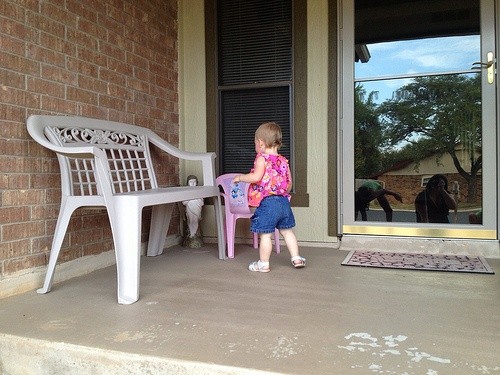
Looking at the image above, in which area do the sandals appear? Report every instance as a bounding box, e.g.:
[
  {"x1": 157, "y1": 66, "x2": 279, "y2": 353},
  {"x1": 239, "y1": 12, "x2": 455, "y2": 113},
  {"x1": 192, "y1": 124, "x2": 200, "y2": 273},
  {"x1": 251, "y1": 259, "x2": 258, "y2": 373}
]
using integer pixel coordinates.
[
  {"x1": 291, "y1": 256, "x2": 305, "y2": 267},
  {"x1": 248, "y1": 260, "x2": 269, "y2": 272}
]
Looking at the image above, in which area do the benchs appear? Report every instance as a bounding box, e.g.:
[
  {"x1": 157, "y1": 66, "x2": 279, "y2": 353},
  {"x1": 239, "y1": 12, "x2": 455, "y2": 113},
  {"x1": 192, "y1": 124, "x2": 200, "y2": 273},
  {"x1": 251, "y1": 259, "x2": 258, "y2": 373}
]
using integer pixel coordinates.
[{"x1": 26, "y1": 115, "x2": 229, "y2": 305}]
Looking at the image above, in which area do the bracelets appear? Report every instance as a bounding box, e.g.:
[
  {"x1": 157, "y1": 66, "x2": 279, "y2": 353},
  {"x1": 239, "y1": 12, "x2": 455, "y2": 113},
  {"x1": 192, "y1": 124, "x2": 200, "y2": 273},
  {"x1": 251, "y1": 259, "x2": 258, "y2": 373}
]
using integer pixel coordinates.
[{"x1": 238, "y1": 175, "x2": 242, "y2": 182}]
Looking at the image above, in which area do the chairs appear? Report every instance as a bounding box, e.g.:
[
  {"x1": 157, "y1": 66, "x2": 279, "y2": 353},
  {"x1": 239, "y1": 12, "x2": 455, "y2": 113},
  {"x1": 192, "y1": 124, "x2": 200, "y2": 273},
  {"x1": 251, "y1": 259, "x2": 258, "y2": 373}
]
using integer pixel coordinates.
[{"x1": 216, "y1": 173, "x2": 281, "y2": 259}]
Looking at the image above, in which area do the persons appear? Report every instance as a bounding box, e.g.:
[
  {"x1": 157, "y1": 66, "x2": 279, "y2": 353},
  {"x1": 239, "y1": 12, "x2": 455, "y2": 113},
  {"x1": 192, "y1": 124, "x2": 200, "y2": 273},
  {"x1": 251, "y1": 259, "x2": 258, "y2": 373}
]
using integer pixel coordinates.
[
  {"x1": 182, "y1": 175, "x2": 204, "y2": 237},
  {"x1": 233, "y1": 123, "x2": 306, "y2": 272},
  {"x1": 355, "y1": 174, "x2": 457, "y2": 224}
]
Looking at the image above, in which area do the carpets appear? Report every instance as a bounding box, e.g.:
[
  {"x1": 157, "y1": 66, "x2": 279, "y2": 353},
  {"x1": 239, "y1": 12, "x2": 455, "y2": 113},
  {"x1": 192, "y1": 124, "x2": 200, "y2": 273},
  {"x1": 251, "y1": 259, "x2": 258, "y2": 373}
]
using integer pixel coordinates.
[{"x1": 341, "y1": 250, "x2": 494, "y2": 275}]
